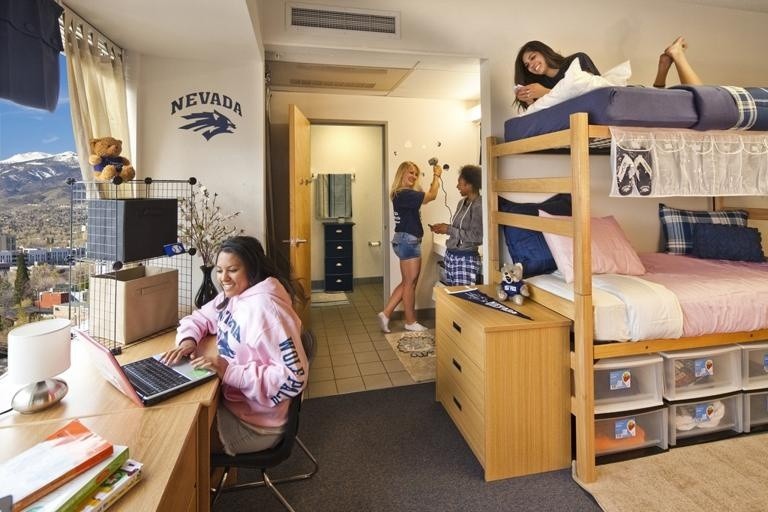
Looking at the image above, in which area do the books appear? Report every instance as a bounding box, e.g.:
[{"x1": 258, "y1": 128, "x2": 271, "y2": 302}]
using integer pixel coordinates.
[{"x1": 1, "y1": 413, "x2": 145, "y2": 512}]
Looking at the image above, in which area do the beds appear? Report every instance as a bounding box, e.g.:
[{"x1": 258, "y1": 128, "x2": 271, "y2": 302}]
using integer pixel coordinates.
[{"x1": 482, "y1": 84, "x2": 767, "y2": 482}]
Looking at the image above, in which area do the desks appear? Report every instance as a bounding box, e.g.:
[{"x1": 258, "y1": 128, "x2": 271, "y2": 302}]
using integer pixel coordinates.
[
  {"x1": 0, "y1": 400, "x2": 205, "y2": 512},
  {"x1": 0, "y1": 312, "x2": 229, "y2": 512}
]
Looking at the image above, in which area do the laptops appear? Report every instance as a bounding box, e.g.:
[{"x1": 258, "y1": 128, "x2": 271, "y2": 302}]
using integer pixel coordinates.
[{"x1": 72, "y1": 327, "x2": 218, "y2": 409}]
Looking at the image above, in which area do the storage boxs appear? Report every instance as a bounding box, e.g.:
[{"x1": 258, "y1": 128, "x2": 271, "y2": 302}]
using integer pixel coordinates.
[
  {"x1": 569, "y1": 345, "x2": 668, "y2": 413},
  {"x1": 78, "y1": 196, "x2": 182, "y2": 262},
  {"x1": 743, "y1": 389, "x2": 767, "y2": 435},
  {"x1": 743, "y1": 342, "x2": 767, "y2": 396},
  {"x1": 575, "y1": 401, "x2": 671, "y2": 458},
  {"x1": 670, "y1": 391, "x2": 746, "y2": 444},
  {"x1": 654, "y1": 345, "x2": 744, "y2": 403},
  {"x1": 85, "y1": 267, "x2": 180, "y2": 345}
]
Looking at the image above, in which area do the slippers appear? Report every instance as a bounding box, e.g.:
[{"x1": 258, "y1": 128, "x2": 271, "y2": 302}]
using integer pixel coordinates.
[{"x1": 615, "y1": 145, "x2": 653, "y2": 195}]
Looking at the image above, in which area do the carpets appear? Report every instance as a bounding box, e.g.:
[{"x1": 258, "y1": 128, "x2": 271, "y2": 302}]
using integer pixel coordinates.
[
  {"x1": 209, "y1": 376, "x2": 606, "y2": 512},
  {"x1": 571, "y1": 429, "x2": 767, "y2": 512},
  {"x1": 309, "y1": 287, "x2": 351, "y2": 308},
  {"x1": 383, "y1": 328, "x2": 440, "y2": 383}
]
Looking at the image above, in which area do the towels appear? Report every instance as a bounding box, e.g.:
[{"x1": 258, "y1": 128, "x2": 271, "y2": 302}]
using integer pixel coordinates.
[{"x1": 314, "y1": 172, "x2": 353, "y2": 220}]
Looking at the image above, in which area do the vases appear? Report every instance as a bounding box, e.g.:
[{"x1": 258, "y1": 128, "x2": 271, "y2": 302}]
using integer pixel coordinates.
[{"x1": 195, "y1": 262, "x2": 218, "y2": 311}]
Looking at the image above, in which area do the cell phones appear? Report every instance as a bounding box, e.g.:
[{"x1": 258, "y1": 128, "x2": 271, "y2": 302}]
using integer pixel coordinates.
[
  {"x1": 516, "y1": 84, "x2": 525, "y2": 91},
  {"x1": 428, "y1": 224, "x2": 433, "y2": 229}
]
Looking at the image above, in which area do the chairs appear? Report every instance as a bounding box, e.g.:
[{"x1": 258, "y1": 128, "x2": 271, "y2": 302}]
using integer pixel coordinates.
[{"x1": 207, "y1": 329, "x2": 320, "y2": 512}]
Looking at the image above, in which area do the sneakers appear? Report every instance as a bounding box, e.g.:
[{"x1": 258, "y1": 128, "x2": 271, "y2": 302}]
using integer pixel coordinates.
[
  {"x1": 404, "y1": 320, "x2": 428, "y2": 332},
  {"x1": 378, "y1": 312, "x2": 391, "y2": 333}
]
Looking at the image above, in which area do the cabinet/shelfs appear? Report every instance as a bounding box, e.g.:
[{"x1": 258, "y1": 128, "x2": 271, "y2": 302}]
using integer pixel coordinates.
[
  {"x1": 430, "y1": 281, "x2": 574, "y2": 482},
  {"x1": 66, "y1": 174, "x2": 196, "y2": 355},
  {"x1": 322, "y1": 220, "x2": 354, "y2": 295}
]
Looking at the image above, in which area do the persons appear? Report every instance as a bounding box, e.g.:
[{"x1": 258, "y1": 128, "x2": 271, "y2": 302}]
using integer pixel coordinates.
[
  {"x1": 157, "y1": 235, "x2": 310, "y2": 479},
  {"x1": 429, "y1": 165, "x2": 482, "y2": 287},
  {"x1": 377, "y1": 160, "x2": 442, "y2": 334},
  {"x1": 507, "y1": 35, "x2": 704, "y2": 110}
]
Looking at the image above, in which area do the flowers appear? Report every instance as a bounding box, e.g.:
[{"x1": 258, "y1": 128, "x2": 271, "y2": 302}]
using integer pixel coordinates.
[{"x1": 172, "y1": 180, "x2": 243, "y2": 269}]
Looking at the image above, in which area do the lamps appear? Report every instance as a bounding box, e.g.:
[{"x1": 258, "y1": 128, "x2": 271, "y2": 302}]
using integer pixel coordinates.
[{"x1": 5, "y1": 316, "x2": 73, "y2": 415}]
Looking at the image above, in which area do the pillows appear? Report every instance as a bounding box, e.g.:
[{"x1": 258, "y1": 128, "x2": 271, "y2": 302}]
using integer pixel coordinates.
[
  {"x1": 495, "y1": 191, "x2": 574, "y2": 280},
  {"x1": 693, "y1": 219, "x2": 766, "y2": 266},
  {"x1": 657, "y1": 202, "x2": 751, "y2": 255},
  {"x1": 518, "y1": 60, "x2": 634, "y2": 117},
  {"x1": 535, "y1": 210, "x2": 649, "y2": 281}
]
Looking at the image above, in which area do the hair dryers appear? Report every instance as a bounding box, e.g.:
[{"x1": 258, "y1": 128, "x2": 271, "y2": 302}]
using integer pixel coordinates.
[{"x1": 428, "y1": 158, "x2": 438, "y2": 167}]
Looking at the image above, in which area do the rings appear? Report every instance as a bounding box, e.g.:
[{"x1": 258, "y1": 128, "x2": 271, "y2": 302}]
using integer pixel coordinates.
[
  {"x1": 527, "y1": 94, "x2": 530, "y2": 98},
  {"x1": 439, "y1": 229, "x2": 440, "y2": 231}
]
